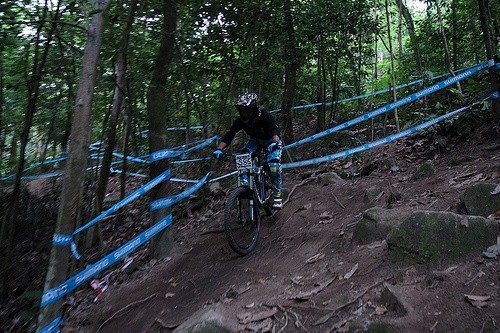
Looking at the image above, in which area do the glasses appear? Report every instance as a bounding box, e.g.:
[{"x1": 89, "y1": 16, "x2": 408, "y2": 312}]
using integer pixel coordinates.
[{"x1": 237, "y1": 106, "x2": 252, "y2": 113}]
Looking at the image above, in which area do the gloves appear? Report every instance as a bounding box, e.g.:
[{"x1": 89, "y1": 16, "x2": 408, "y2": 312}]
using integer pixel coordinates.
[
  {"x1": 262, "y1": 139, "x2": 281, "y2": 154},
  {"x1": 213, "y1": 150, "x2": 225, "y2": 160}
]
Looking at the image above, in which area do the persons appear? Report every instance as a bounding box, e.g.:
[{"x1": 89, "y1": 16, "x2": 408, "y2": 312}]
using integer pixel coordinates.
[{"x1": 210, "y1": 92, "x2": 285, "y2": 209}]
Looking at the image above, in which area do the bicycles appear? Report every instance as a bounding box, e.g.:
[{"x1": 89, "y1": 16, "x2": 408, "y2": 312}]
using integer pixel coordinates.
[{"x1": 216, "y1": 140, "x2": 278, "y2": 255}]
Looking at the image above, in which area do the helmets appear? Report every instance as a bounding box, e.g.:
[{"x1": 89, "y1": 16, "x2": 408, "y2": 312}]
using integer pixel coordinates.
[{"x1": 234, "y1": 92, "x2": 262, "y2": 125}]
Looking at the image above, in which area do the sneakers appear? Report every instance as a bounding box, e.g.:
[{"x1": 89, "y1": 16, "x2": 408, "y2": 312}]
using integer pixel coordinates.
[{"x1": 270, "y1": 197, "x2": 283, "y2": 209}]
